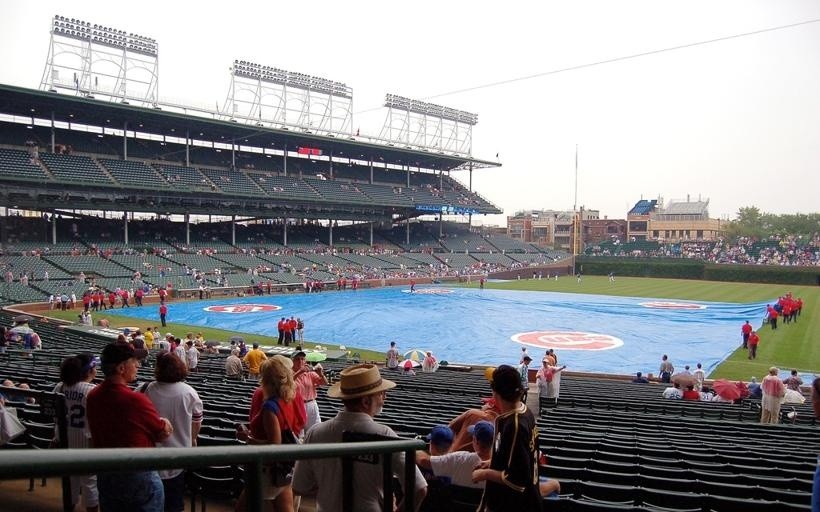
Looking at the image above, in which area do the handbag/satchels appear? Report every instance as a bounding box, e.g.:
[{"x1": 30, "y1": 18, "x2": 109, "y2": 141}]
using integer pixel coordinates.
[
  {"x1": 0, "y1": 402, "x2": 28, "y2": 445},
  {"x1": 263, "y1": 399, "x2": 300, "y2": 487}
]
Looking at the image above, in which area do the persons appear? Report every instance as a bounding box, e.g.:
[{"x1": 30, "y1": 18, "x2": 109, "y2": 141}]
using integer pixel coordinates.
[
  {"x1": 201, "y1": 175, "x2": 228, "y2": 189},
  {"x1": 592, "y1": 232, "x2": 820, "y2": 266},
  {"x1": 291, "y1": 172, "x2": 480, "y2": 205},
  {"x1": 0, "y1": 302, "x2": 567, "y2": 512},
  {"x1": 741, "y1": 320, "x2": 760, "y2": 360},
  {"x1": 518, "y1": 271, "x2": 616, "y2": 282},
  {"x1": 765, "y1": 292, "x2": 802, "y2": 330},
  {"x1": 223, "y1": 151, "x2": 283, "y2": 191},
  {"x1": 48, "y1": 143, "x2": 72, "y2": 154},
  {"x1": 633, "y1": 355, "x2": 802, "y2": 424},
  {"x1": 1, "y1": 211, "x2": 557, "y2": 312},
  {"x1": 28, "y1": 142, "x2": 39, "y2": 164},
  {"x1": 144, "y1": 159, "x2": 180, "y2": 181},
  {"x1": 811, "y1": 378, "x2": 820, "y2": 512}
]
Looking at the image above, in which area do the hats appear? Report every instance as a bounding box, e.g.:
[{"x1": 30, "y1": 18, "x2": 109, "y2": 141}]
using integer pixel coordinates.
[
  {"x1": 486, "y1": 365, "x2": 523, "y2": 393},
  {"x1": 426, "y1": 425, "x2": 454, "y2": 448},
  {"x1": 480, "y1": 398, "x2": 499, "y2": 411},
  {"x1": 524, "y1": 356, "x2": 532, "y2": 361},
  {"x1": 100, "y1": 343, "x2": 149, "y2": 367},
  {"x1": 291, "y1": 350, "x2": 306, "y2": 360},
  {"x1": 327, "y1": 363, "x2": 398, "y2": 400},
  {"x1": 542, "y1": 359, "x2": 549, "y2": 362},
  {"x1": 89, "y1": 355, "x2": 101, "y2": 370},
  {"x1": 467, "y1": 423, "x2": 495, "y2": 443}
]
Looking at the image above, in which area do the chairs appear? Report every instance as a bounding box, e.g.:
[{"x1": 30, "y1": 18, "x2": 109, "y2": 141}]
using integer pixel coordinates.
[
  {"x1": 0, "y1": 307, "x2": 820, "y2": 510},
  {"x1": 0, "y1": 142, "x2": 571, "y2": 305}
]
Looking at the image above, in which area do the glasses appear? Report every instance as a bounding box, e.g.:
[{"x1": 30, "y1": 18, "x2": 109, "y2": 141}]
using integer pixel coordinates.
[{"x1": 542, "y1": 362, "x2": 547, "y2": 364}]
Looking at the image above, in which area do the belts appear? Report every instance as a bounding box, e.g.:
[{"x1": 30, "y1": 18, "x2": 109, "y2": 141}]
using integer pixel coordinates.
[{"x1": 304, "y1": 399, "x2": 314, "y2": 403}]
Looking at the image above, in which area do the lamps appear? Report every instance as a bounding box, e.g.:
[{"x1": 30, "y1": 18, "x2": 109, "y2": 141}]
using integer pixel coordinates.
[
  {"x1": 51, "y1": 15, "x2": 157, "y2": 59},
  {"x1": 383, "y1": 93, "x2": 478, "y2": 127},
  {"x1": 232, "y1": 58, "x2": 352, "y2": 101}
]
